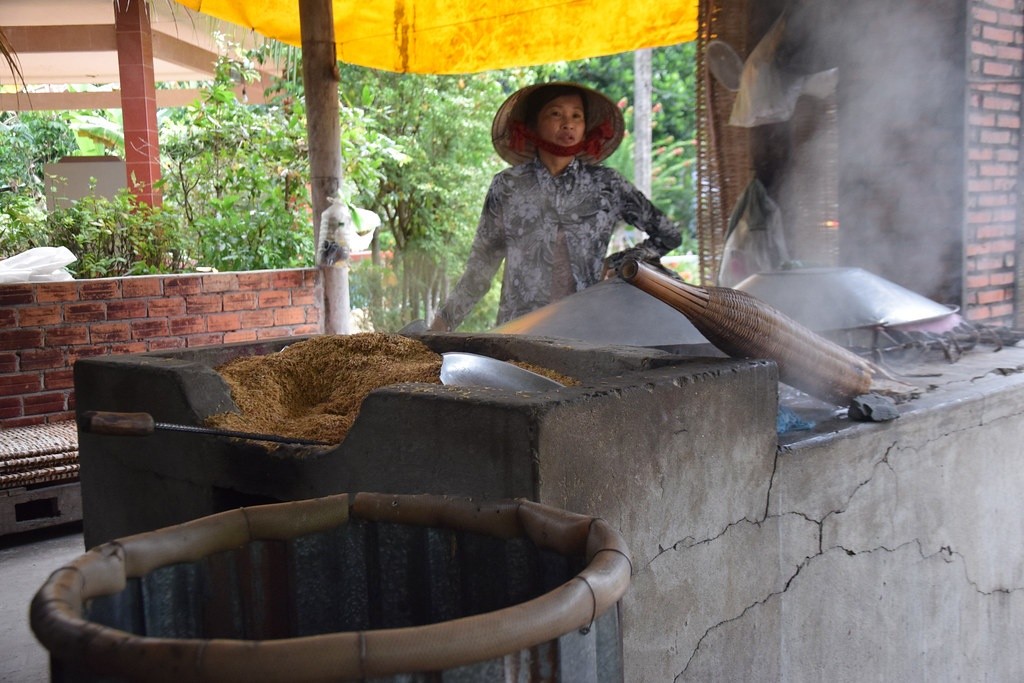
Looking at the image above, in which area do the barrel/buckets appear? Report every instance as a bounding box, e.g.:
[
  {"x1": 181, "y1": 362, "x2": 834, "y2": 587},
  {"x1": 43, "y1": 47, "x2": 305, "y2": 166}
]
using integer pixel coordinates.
[{"x1": 350, "y1": 209, "x2": 381, "y2": 255}]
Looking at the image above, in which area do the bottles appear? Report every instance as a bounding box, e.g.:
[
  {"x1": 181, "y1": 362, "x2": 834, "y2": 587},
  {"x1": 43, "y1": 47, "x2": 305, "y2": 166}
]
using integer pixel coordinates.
[
  {"x1": 334, "y1": 222, "x2": 351, "y2": 261},
  {"x1": 316, "y1": 197, "x2": 353, "y2": 267}
]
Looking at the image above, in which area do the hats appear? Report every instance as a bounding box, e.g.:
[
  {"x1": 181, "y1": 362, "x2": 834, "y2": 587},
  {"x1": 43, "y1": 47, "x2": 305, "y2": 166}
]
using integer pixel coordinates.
[{"x1": 492, "y1": 82, "x2": 625, "y2": 166}]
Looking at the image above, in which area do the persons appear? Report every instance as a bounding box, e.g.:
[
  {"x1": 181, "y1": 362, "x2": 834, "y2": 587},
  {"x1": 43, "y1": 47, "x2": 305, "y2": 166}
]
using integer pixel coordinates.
[{"x1": 429, "y1": 83, "x2": 685, "y2": 336}]
